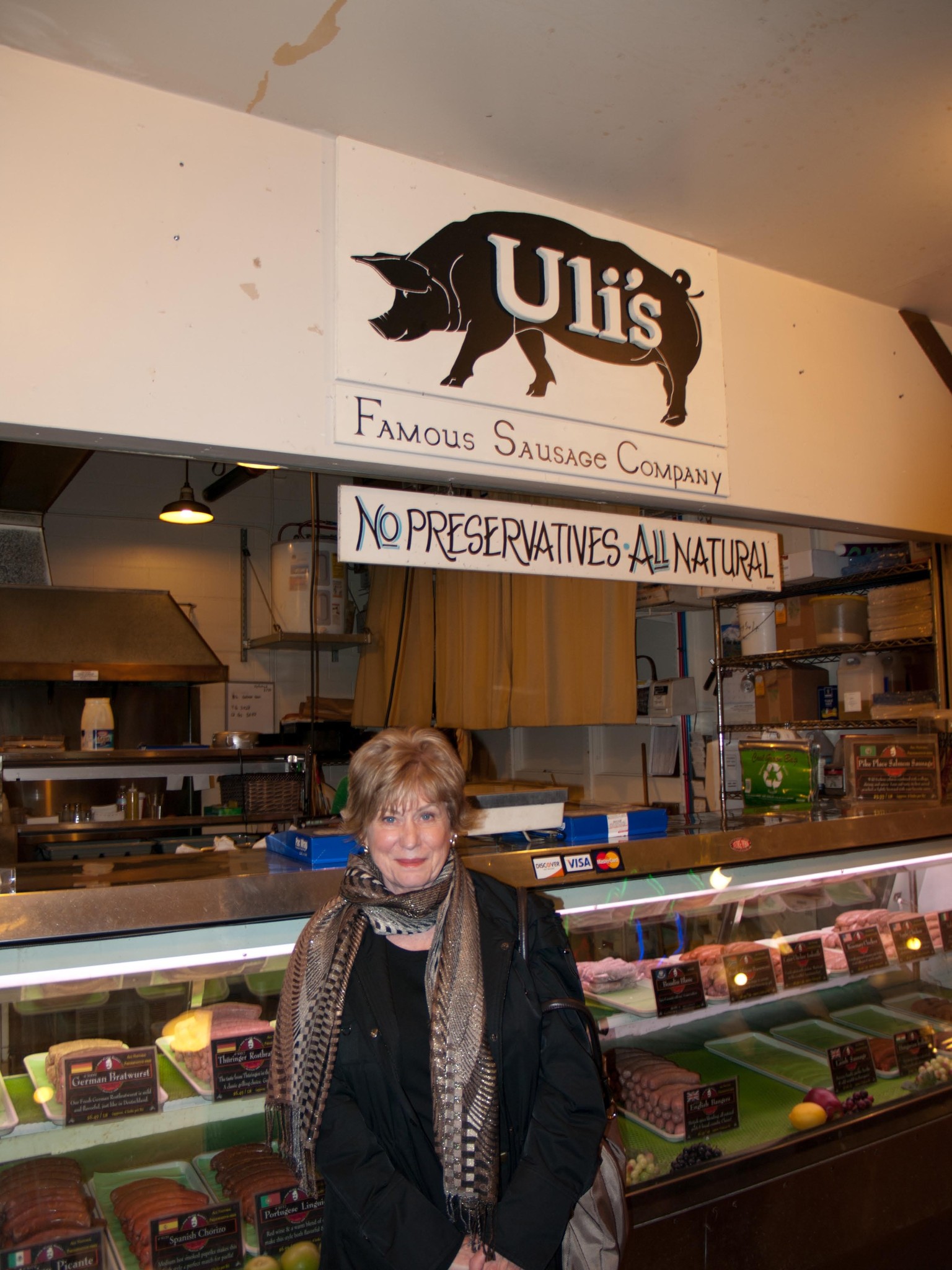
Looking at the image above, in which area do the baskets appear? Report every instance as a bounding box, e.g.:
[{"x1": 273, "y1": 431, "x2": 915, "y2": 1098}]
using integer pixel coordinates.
[{"x1": 216, "y1": 772, "x2": 304, "y2": 815}]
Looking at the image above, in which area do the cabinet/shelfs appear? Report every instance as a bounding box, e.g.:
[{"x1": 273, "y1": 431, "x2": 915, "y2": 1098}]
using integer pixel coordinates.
[{"x1": 0, "y1": 793, "x2": 950, "y2": 1268}]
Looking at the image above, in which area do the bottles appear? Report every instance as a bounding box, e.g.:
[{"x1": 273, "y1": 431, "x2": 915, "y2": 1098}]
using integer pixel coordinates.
[
  {"x1": 81, "y1": 698, "x2": 115, "y2": 751},
  {"x1": 116, "y1": 783, "x2": 139, "y2": 821},
  {"x1": 61, "y1": 802, "x2": 91, "y2": 823}
]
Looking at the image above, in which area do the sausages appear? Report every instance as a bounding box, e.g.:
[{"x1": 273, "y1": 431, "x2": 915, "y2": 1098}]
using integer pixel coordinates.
[{"x1": 1, "y1": 905, "x2": 949, "y2": 1270}]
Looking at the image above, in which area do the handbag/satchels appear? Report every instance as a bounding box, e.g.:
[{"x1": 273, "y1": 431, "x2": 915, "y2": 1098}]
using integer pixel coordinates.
[{"x1": 561, "y1": 1096, "x2": 630, "y2": 1270}]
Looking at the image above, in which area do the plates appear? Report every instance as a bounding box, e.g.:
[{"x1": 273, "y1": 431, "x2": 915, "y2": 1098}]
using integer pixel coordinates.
[{"x1": 4, "y1": 740, "x2": 65, "y2": 753}]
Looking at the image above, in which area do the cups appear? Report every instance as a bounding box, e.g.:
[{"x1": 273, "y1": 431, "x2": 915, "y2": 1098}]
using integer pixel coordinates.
[{"x1": 138, "y1": 792, "x2": 145, "y2": 820}]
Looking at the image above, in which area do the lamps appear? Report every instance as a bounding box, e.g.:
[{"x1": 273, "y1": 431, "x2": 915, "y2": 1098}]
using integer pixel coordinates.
[{"x1": 159, "y1": 457, "x2": 217, "y2": 530}]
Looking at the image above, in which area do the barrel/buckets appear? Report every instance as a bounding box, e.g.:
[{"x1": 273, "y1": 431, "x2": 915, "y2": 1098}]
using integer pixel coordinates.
[
  {"x1": 838, "y1": 650, "x2": 905, "y2": 721},
  {"x1": 740, "y1": 601, "x2": 776, "y2": 655},
  {"x1": 761, "y1": 729, "x2": 867, "y2": 794}
]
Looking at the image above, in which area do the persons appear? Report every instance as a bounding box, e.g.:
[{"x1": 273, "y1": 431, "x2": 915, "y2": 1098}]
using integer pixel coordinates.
[{"x1": 262, "y1": 723, "x2": 609, "y2": 1270}]
